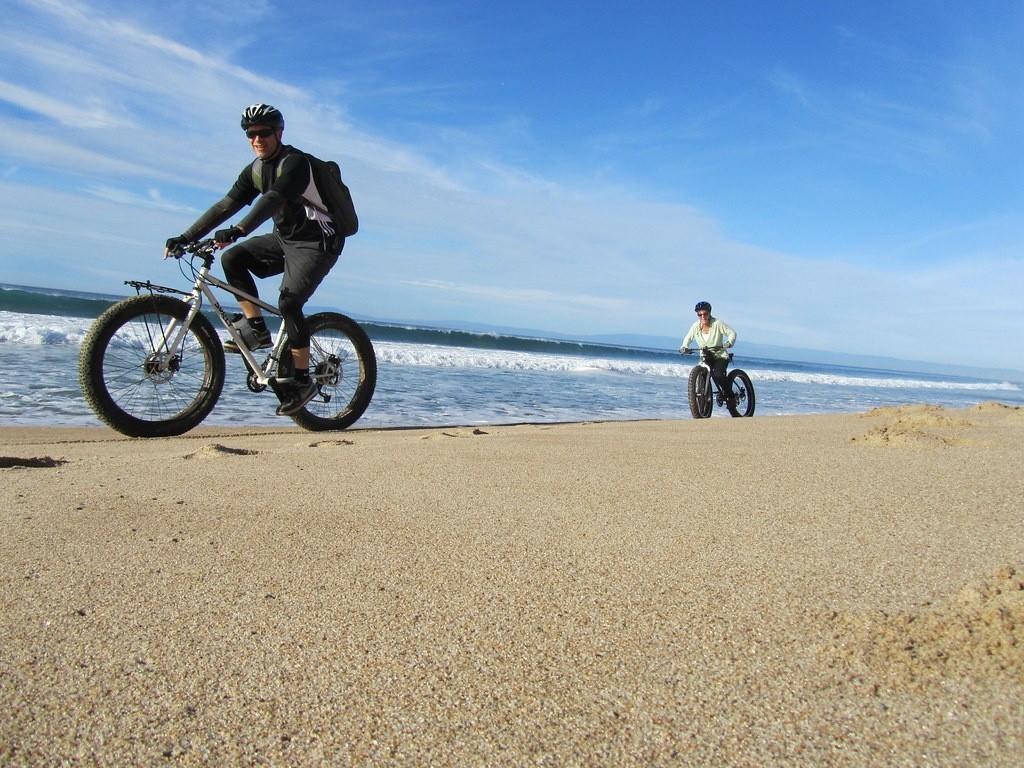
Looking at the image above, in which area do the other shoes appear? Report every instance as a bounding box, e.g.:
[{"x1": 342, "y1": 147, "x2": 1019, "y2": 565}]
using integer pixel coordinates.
[{"x1": 727, "y1": 392, "x2": 736, "y2": 410}]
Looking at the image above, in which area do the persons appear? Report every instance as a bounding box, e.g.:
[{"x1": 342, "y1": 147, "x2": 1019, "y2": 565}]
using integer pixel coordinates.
[
  {"x1": 680, "y1": 301, "x2": 737, "y2": 409},
  {"x1": 163, "y1": 104, "x2": 345, "y2": 416}
]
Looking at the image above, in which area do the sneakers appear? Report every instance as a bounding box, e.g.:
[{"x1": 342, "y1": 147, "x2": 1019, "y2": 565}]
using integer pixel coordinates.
[
  {"x1": 276, "y1": 378, "x2": 318, "y2": 415},
  {"x1": 223, "y1": 328, "x2": 274, "y2": 350}
]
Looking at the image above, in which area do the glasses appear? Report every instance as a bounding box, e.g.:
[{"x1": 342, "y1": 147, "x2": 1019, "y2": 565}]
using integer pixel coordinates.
[
  {"x1": 246, "y1": 128, "x2": 278, "y2": 139},
  {"x1": 697, "y1": 311, "x2": 709, "y2": 317}
]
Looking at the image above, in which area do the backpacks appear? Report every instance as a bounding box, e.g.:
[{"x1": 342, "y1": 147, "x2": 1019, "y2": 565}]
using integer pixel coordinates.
[{"x1": 252, "y1": 145, "x2": 359, "y2": 237}]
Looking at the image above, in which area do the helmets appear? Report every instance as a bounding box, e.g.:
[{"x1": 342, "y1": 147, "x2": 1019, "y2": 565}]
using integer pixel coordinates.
[
  {"x1": 694, "y1": 301, "x2": 711, "y2": 312},
  {"x1": 241, "y1": 103, "x2": 285, "y2": 130}
]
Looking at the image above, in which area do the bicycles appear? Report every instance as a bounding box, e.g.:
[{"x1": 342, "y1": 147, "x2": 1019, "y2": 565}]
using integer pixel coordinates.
[
  {"x1": 679, "y1": 344, "x2": 755, "y2": 419},
  {"x1": 79, "y1": 235, "x2": 378, "y2": 438}
]
